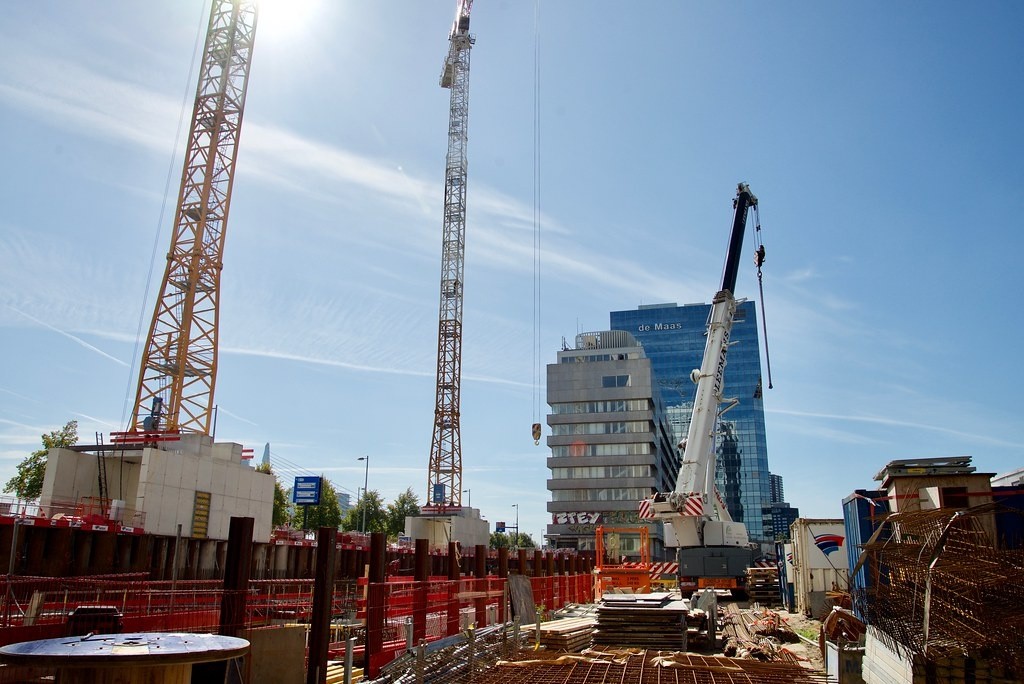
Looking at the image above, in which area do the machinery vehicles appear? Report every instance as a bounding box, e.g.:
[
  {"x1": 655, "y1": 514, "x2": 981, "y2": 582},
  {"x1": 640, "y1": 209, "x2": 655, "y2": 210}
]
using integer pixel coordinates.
[{"x1": 634, "y1": 181, "x2": 776, "y2": 601}]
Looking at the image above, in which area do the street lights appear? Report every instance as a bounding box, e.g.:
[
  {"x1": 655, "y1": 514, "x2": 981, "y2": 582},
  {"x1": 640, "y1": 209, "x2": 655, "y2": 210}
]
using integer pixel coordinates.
[
  {"x1": 512, "y1": 504, "x2": 520, "y2": 548},
  {"x1": 541, "y1": 527, "x2": 545, "y2": 549},
  {"x1": 358, "y1": 456, "x2": 369, "y2": 535},
  {"x1": 463, "y1": 489, "x2": 472, "y2": 508}
]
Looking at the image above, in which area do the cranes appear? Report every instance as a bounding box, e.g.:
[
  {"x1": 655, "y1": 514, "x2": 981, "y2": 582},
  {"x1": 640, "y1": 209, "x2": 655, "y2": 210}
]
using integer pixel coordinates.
[
  {"x1": 422, "y1": 1, "x2": 480, "y2": 510},
  {"x1": 119, "y1": 0, "x2": 264, "y2": 449}
]
